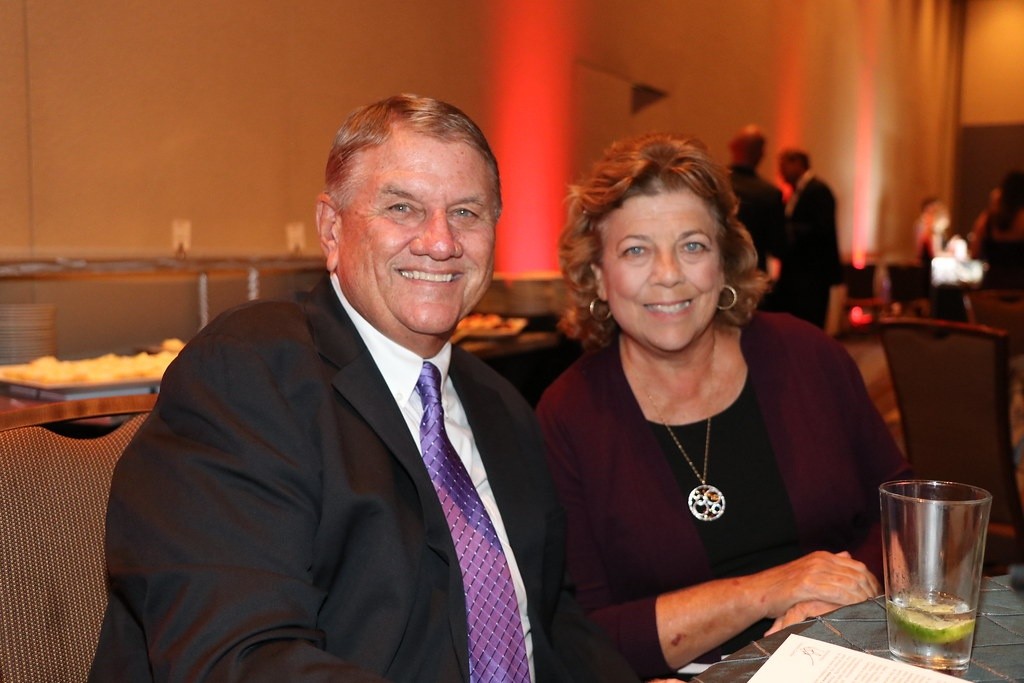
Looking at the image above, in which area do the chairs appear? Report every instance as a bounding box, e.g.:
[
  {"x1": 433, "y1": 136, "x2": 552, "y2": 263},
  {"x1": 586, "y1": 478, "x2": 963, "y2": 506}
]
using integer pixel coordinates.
[
  {"x1": 833, "y1": 290, "x2": 1024, "y2": 577},
  {"x1": 0, "y1": 394, "x2": 160, "y2": 683}
]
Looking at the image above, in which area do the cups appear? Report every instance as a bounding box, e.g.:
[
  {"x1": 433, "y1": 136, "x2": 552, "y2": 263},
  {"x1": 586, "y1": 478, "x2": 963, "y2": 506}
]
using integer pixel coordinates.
[{"x1": 880, "y1": 479, "x2": 993, "y2": 676}]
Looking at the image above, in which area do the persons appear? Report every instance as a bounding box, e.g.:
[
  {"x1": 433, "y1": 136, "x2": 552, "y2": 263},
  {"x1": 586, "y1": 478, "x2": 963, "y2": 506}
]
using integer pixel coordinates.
[
  {"x1": 534, "y1": 132, "x2": 906, "y2": 682},
  {"x1": 777, "y1": 146, "x2": 848, "y2": 338},
  {"x1": 721, "y1": 126, "x2": 785, "y2": 315},
  {"x1": 84, "y1": 92, "x2": 566, "y2": 683},
  {"x1": 916, "y1": 168, "x2": 1024, "y2": 289}
]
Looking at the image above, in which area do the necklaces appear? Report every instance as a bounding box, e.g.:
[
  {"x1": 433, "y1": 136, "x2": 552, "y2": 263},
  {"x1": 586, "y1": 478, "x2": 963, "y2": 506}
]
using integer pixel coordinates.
[{"x1": 633, "y1": 342, "x2": 726, "y2": 522}]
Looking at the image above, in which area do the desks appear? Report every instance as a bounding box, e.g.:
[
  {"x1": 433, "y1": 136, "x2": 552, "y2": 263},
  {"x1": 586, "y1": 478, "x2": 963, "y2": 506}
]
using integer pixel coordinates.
[{"x1": 688, "y1": 573, "x2": 1024, "y2": 683}]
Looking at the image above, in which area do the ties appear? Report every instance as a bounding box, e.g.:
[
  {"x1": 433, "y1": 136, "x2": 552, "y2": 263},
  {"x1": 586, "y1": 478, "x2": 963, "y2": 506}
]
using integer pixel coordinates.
[{"x1": 417, "y1": 361, "x2": 530, "y2": 683}]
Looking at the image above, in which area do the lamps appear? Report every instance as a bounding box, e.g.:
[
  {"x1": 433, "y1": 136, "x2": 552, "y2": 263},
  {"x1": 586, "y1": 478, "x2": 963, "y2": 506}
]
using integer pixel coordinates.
[{"x1": 568, "y1": 57, "x2": 669, "y2": 116}]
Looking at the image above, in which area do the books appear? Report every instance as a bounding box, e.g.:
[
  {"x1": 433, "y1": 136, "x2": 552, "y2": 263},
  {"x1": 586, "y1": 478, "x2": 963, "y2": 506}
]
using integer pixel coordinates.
[{"x1": 746, "y1": 633, "x2": 972, "y2": 683}]
